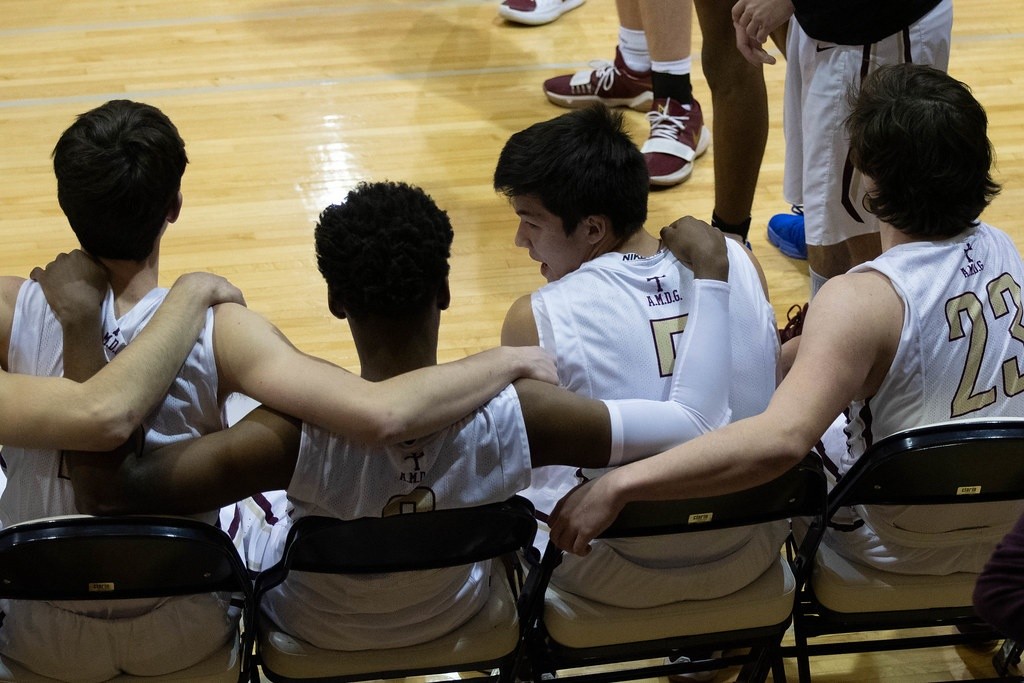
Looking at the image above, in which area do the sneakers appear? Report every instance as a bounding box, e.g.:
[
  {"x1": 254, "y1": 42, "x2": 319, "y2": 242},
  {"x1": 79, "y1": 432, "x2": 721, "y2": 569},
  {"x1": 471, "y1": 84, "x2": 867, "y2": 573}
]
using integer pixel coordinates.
[
  {"x1": 543, "y1": 46, "x2": 654, "y2": 111},
  {"x1": 499, "y1": 0, "x2": 586, "y2": 25},
  {"x1": 667, "y1": 650, "x2": 724, "y2": 682},
  {"x1": 637, "y1": 94, "x2": 710, "y2": 187},
  {"x1": 766, "y1": 205, "x2": 808, "y2": 259},
  {"x1": 775, "y1": 303, "x2": 809, "y2": 342}
]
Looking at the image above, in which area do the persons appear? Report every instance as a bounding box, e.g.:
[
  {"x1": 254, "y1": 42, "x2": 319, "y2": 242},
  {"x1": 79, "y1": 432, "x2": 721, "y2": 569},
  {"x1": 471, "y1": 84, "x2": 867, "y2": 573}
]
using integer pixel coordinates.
[
  {"x1": 30, "y1": 180, "x2": 732, "y2": 652},
  {"x1": 494, "y1": 103, "x2": 793, "y2": 683},
  {"x1": 972, "y1": 512, "x2": 1024, "y2": 644},
  {"x1": 0, "y1": 272, "x2": 249, "y2": 452},
  {"x1": 547, "y1": 62, "x2": 1024, "y2": 575},
  {"x1": 0, "y1": 99, "x2": 560, "y2": 683},
  {"x1": 497, "y1": 0, "x2": 954, "y2": 344}
]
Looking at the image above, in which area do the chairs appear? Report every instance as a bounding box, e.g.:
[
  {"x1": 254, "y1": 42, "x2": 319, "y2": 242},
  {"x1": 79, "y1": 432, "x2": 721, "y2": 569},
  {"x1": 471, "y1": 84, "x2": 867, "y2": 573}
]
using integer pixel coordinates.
[{"x1": 0, "y1": 415, "x2": 1024, "y2": 683}]
